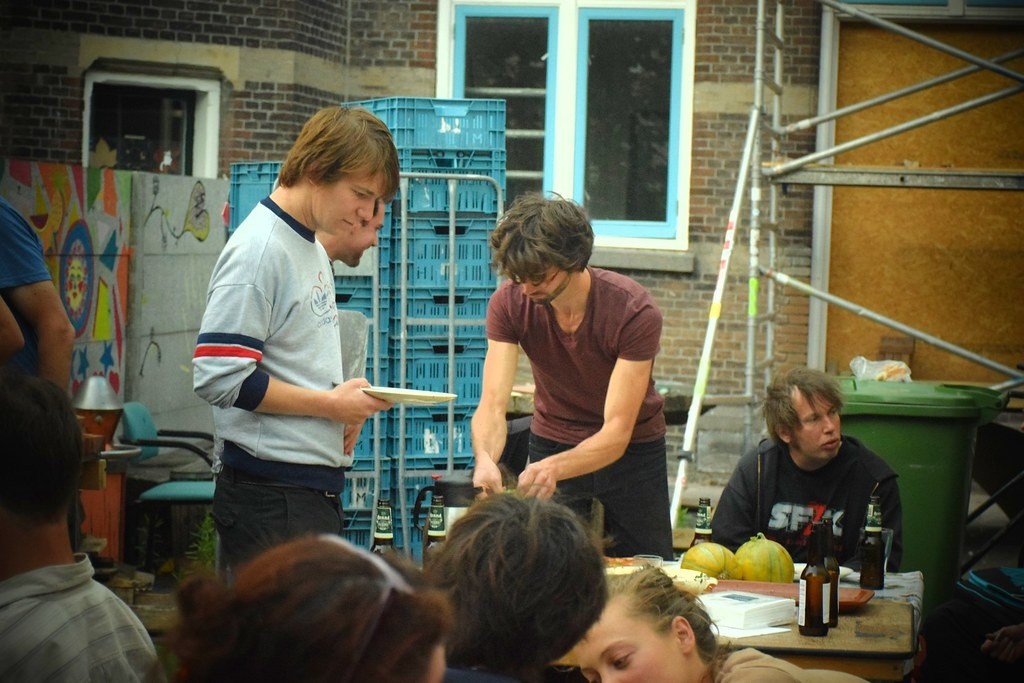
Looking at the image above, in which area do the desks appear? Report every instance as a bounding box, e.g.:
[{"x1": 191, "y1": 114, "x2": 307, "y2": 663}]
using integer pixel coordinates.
[{"x1": 550, "y1": 558, "x2": 923, "y2": 683}]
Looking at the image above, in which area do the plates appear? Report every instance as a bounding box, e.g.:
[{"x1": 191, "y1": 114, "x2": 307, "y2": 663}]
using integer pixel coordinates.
[
  {"x1": 712, "y1": 576, "x2": 876, "y2": 613},
  {"x1": 361, "y1": 386, "x2": 458, "y2": 406}
]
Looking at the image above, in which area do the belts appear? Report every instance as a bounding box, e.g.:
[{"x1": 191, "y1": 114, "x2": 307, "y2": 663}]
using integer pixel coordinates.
[{"x1": 219, "y1": 469, "x2": 342, "y2": 497}]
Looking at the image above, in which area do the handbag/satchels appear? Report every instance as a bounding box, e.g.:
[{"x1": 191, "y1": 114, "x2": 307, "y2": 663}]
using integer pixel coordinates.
[{"x1": 850, "y1": 356, "x2": 913, "y2": 383}]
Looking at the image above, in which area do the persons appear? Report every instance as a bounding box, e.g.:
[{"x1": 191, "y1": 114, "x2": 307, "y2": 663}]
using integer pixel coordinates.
[
  {"x1": 577, "y1": 568, "x2": 869, "y2": 683},
  {"x1": 0, "y1": 196, "x2": 75, "y2": 395},
  {"x1": 469, "y1": 193, "x2": 672, "y2": 560},
  {"x1": 312, "y1": 199, "x2": 385, "y2": 279},
  {"x1": 914, "y1": 598, "x2": 1024, "y2": 683},
  {"x1": 193, "y1": 105, "x2": 400, "y2": 580},
  {"x1": 0, "y1": 371, "x2": 164, "y2": 683},
  {"x1": 418, "y1": 492, "x2": 609, "y2": 683},
  {"x1": 159, "y1": 535, "x2": 449, "y2": 683},
  {"x1": 710, "y1": 365, "x2": 902, "y2": 569}
]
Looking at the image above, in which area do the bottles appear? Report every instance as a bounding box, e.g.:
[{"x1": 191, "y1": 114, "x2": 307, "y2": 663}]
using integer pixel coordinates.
[
  {"x1": 690, "y1": 497, "x2": 713, "y2": 547},
  {"x1": 821, "y1": 515, "x2": 840, "y2": 628},
  {"x1": 799, "y1": 521, "x2": 831, "y2": 637},
  {"x1": 422, "y1": 495, "x2": 447, "y2": 571},
  {"x1": 860, "y1": 495, "x2": 884, "y2": 590},
  {"x1": 369, "y1": 498, "x2": 401, "y2": 557}
]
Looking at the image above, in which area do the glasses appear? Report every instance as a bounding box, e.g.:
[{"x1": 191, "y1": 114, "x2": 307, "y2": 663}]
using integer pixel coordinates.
[{"x1": 507, "y1": 267, "x2": 560, "y2": 287}]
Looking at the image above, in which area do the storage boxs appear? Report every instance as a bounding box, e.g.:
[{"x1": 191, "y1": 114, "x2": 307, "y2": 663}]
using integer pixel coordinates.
[{"x1": 228, "y1": 97, "x2": 507, "y2": 570}]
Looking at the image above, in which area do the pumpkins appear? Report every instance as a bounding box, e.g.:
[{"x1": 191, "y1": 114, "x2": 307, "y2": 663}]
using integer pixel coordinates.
[{"x1": 682, "y1": 533, "x2": 796, "y2": 583}]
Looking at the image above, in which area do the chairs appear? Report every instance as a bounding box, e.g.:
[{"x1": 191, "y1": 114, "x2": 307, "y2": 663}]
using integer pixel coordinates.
[{"x1": 121, "y1": 401, "x2": 215, "y2": 569}]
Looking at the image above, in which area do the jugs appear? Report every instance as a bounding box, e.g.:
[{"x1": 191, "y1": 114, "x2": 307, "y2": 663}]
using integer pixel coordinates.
[{"x1": 414, "y1": 476, "x2": 484, "y2": 547}]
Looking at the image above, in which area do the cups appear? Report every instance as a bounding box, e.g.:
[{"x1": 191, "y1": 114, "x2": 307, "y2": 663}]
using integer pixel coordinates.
[
  {"x1": 858, "y1": 527, "x2": 894, "y2": 576},
  {"x1": 634, "y1": 554, "x2": 664, "y2": 569}
]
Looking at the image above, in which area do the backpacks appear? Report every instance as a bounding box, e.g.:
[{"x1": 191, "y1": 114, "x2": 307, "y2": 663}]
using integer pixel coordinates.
[
  {"x1": 957, "y1": 567, "x2": 1024, "y2": 625},
  {"x1": 922, "y1": 599, "x2": 1024, "y2": 683}
]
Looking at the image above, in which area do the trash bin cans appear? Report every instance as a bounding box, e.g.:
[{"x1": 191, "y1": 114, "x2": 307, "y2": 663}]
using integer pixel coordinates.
[{"x1": 833, "y1": 374, "x2": 1011, "y2": 599}]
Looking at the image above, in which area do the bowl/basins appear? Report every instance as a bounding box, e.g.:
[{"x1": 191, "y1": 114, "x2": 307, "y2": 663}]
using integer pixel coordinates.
[{"x1": 604, "y1": 567, "x2": 708, "y2": 596}]
